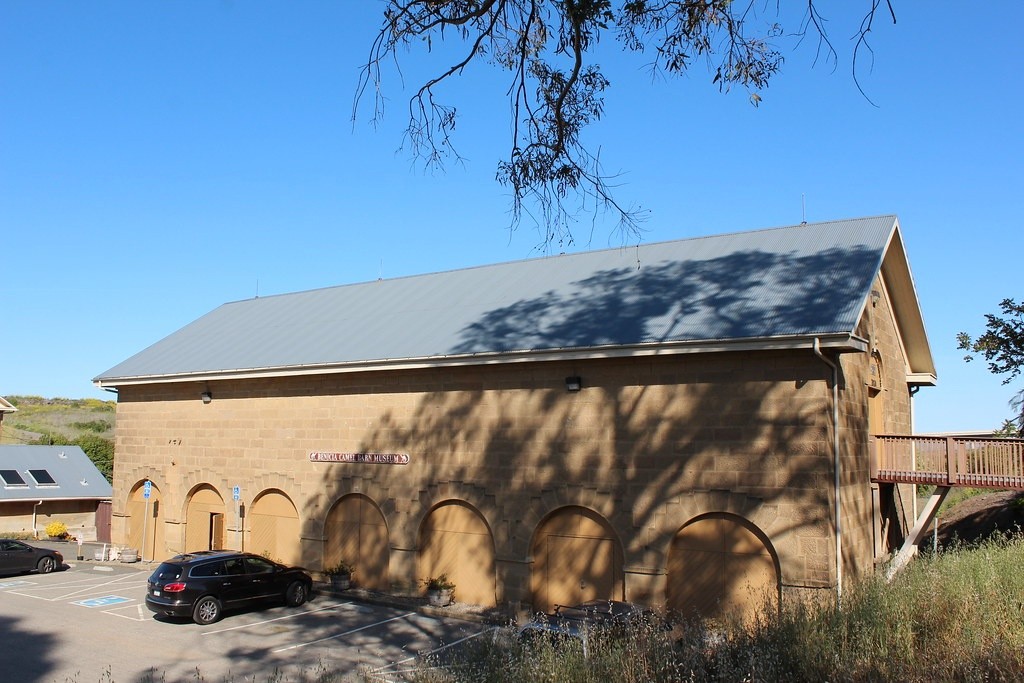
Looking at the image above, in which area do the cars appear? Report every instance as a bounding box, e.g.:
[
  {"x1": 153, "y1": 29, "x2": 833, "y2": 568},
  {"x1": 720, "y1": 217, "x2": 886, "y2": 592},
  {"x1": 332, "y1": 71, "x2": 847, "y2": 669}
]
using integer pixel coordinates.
[{"x1": 0, "y1": 539, "x2": 64, "y2": 578}]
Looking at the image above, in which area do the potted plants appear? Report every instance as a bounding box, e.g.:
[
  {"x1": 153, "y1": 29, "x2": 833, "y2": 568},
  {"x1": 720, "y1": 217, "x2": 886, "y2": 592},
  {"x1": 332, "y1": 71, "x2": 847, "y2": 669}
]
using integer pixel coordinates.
[
  {"x1": 326, "y1": 562, "x2": 355, "y2": 591},
  {"x1": 423, "y1": 574, "x2": 454, "y2": 607}
]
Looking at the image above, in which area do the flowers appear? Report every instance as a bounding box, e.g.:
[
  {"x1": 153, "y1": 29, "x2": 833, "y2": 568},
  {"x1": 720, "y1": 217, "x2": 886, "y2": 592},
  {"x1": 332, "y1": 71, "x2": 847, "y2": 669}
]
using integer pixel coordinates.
[{"x1": 45, "y1": 522, "x2": 67, "y2": 537}]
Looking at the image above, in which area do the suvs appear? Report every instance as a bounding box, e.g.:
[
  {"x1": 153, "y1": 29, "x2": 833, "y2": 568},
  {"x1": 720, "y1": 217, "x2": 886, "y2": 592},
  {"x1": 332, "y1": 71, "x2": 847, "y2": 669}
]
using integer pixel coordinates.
[{"x1": 145, "y1": 549, "x2": 313, "y2": 619}]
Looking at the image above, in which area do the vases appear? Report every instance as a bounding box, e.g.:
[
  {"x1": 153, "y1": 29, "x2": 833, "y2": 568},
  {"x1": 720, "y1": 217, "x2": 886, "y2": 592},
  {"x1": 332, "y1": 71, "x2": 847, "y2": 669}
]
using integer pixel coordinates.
[{"x1": 49, "y1": 534, "x2": 62, "y2": 541}]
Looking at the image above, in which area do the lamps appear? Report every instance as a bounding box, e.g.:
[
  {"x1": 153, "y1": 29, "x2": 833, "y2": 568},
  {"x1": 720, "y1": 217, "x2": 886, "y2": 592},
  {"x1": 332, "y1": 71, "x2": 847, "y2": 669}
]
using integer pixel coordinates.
[
  {"x1": 565, "y1": 376, "x2": 582, "y2": 391},
  {"x1": 202, "y1": 392, "x2": 212, "y2": 403},
  {"x1": 872, "y1": 290, "x2": 881, "y2": 302}
]
